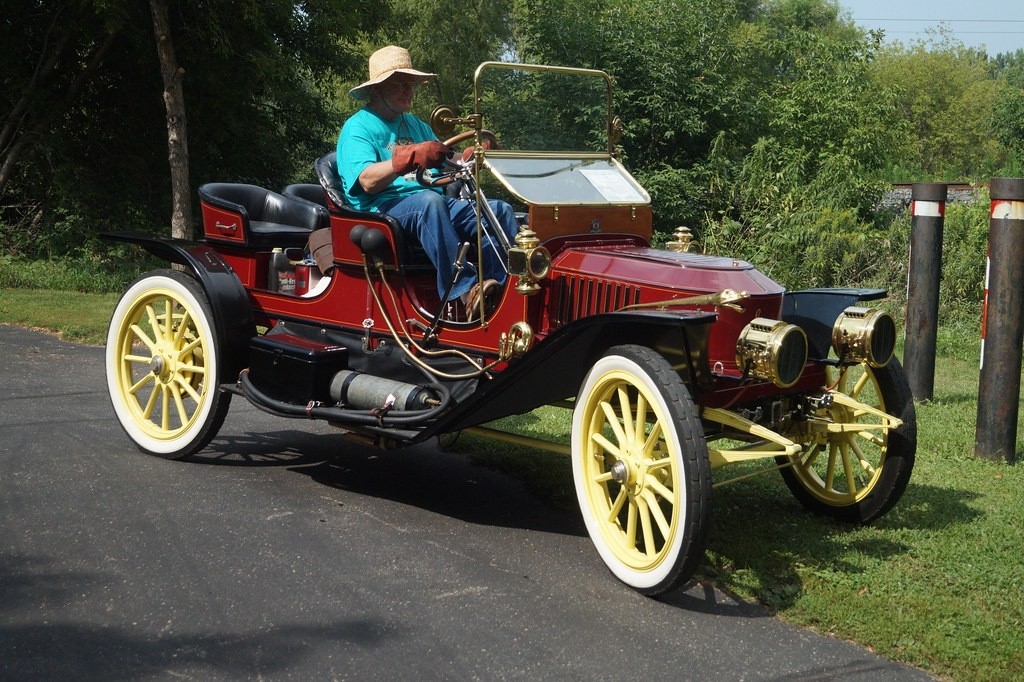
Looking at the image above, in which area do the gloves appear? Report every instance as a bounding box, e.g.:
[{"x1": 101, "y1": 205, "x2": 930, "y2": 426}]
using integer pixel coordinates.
[
  {"x1": 392, "y1": 140, "x2": 454, "y2": 177},
  {"x1": 463, "y1": 140, "x2": 499, "y2": 162}
]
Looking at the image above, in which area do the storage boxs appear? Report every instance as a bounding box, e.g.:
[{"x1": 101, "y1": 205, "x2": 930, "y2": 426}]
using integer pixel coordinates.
[{"x1": 249, "y1": 333, "x2": 349, "y2": 409}]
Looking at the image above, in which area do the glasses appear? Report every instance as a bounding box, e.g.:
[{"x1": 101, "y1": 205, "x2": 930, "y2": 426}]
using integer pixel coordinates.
[{"x1": 386, "y1": 79, "x2": 419, "y2": 93}]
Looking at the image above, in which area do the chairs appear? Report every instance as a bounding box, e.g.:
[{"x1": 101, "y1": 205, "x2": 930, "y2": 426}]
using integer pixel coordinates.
[
  {"x1": 282, "y1": 181, "x2": 335, "y2": 226},
  {"x1": 198, "y1": 182, "x2": 321, "y2": 246}
]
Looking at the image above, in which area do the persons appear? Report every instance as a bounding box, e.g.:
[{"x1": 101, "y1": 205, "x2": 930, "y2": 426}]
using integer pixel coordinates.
[{"x1": 335, "y1": 44, "x2": 520, "y2": 322}]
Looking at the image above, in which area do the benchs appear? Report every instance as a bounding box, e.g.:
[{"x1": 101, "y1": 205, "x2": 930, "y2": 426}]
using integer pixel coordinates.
[{"x1": 316, "y1": 150, "x2": 529, "y2": 267}]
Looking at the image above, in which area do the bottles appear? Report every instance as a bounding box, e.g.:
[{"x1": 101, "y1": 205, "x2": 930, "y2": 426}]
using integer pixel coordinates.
[{"x1": 268, "y1": 248, "x2": 289, "y2": 295}]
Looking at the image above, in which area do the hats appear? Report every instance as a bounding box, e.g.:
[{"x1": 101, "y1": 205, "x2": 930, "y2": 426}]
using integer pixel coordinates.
[{"x1": 349, "y1": 44, "x2": 438, "y2": 100}]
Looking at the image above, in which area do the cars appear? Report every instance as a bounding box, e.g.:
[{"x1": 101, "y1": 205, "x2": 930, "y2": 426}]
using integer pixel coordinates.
[{"x1": 107, "y1": 62, "x2": 919, "y2": 597}]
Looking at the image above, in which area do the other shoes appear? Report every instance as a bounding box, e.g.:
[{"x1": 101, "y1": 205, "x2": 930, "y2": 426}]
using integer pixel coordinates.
[{"x1": 465, "y1": 279, "x2": 499, "y2": 322}]
